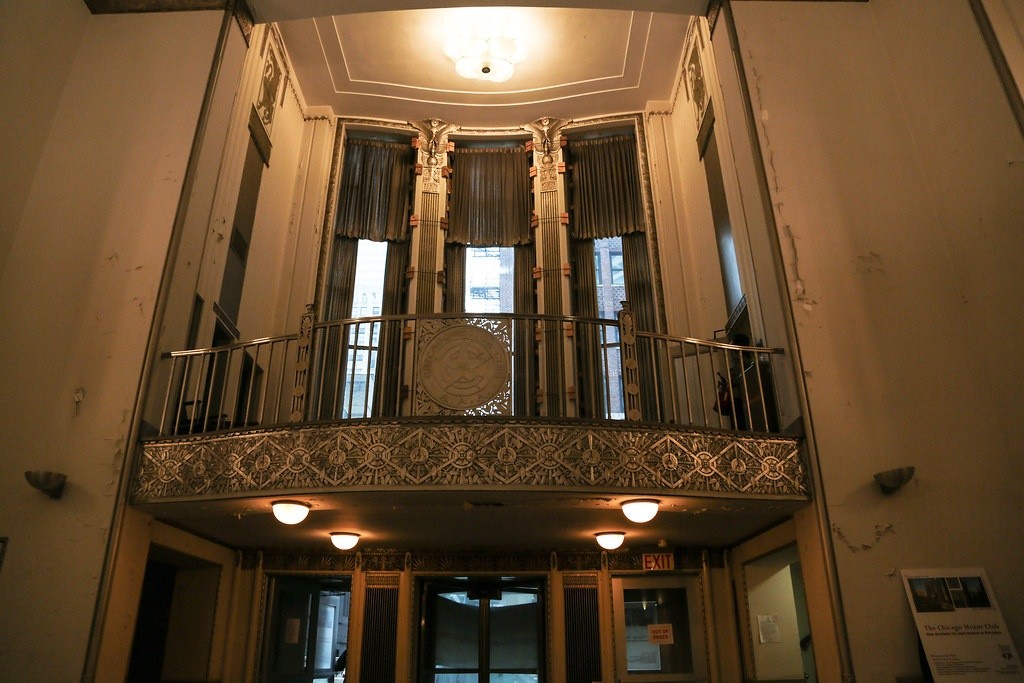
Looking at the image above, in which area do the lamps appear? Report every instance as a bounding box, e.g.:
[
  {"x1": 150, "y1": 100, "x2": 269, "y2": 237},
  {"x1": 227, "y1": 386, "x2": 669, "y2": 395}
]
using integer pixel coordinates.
[
  {"x1": 596, "y1": 534, "x2": 624, "y2": 549},
  {"x1": 331, "y1": 534, "x2": 359, "y2": 550},
  {"x1": 272, "y1": 504, "x2": 309, "y2": 525},
  {"x1": 622, "y1": 500, "x2": 659, "y2": 523}
]
